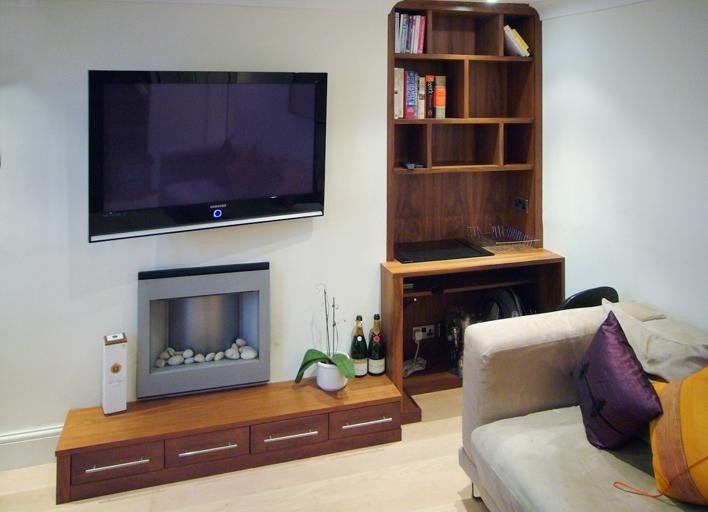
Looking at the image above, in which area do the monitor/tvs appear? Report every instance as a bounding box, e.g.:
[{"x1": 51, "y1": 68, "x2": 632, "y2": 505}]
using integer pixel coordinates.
[{"x1": 88, "y1": 70, "x2": 328, "y2": 241}]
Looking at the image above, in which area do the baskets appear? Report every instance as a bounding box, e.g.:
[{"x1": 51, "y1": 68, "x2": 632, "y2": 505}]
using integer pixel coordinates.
[{"x1": 463, "y1": 223, "x2": 541, "y2": 254}]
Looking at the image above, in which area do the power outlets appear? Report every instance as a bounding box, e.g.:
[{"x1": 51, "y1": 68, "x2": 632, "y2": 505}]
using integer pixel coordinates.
[{"x1": 411, "y1": 325, "x2": 434, "y2": 340}]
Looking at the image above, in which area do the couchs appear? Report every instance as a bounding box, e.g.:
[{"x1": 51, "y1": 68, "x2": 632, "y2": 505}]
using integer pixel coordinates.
[{"x1": 452, "y1": 302, "x2": 707, "y2": 512}]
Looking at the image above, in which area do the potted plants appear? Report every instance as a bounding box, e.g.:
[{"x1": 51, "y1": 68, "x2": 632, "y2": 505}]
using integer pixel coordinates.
[{"x1": 295, "y1": 283, "x2": 352, "y2": 390}]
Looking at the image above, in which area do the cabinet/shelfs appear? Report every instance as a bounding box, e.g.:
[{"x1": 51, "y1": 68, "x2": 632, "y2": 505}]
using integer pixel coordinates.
[
  {"x1": 387, "y1": 1, "x2": 546, "y2": 256},
  {"x1": 52, "y1": 371, "x2": 403, "y2": 506},
  {"x1": 379, "y1": 246, "x2": 566, "y2": 397}
]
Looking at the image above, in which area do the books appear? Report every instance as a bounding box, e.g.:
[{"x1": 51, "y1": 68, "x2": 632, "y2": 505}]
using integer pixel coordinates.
[{"x1": 393, "y1": 11, "x2": 533, "y2": 121}]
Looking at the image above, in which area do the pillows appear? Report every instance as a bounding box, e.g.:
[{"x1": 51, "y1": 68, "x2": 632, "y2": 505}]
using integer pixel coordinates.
[
  {"x1": 569, "y1": 312, "x2": 654, "y2": 446},
  {"x1": 646, "y1": 367, "x2": 707, "y2": 507},
  {"x1": 607, "y1": 301, "x2": 708, "y2": 380}
]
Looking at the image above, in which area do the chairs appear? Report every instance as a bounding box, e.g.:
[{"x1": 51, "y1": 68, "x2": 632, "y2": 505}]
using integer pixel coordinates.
[{"x1": 554, "y1": 286, "x2": 618, "y2": 313}]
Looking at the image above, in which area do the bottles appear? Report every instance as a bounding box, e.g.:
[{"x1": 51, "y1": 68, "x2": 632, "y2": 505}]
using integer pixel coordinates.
[
  {"x1": 367, "y1": 314, "x2": 386, "y2": 377},
  {"x1": 447, "y1": 327, "x2": 461, "y2": 375},
  {"x1": 349, "y1": 315, "x2": 366, "y2": 378}
]
